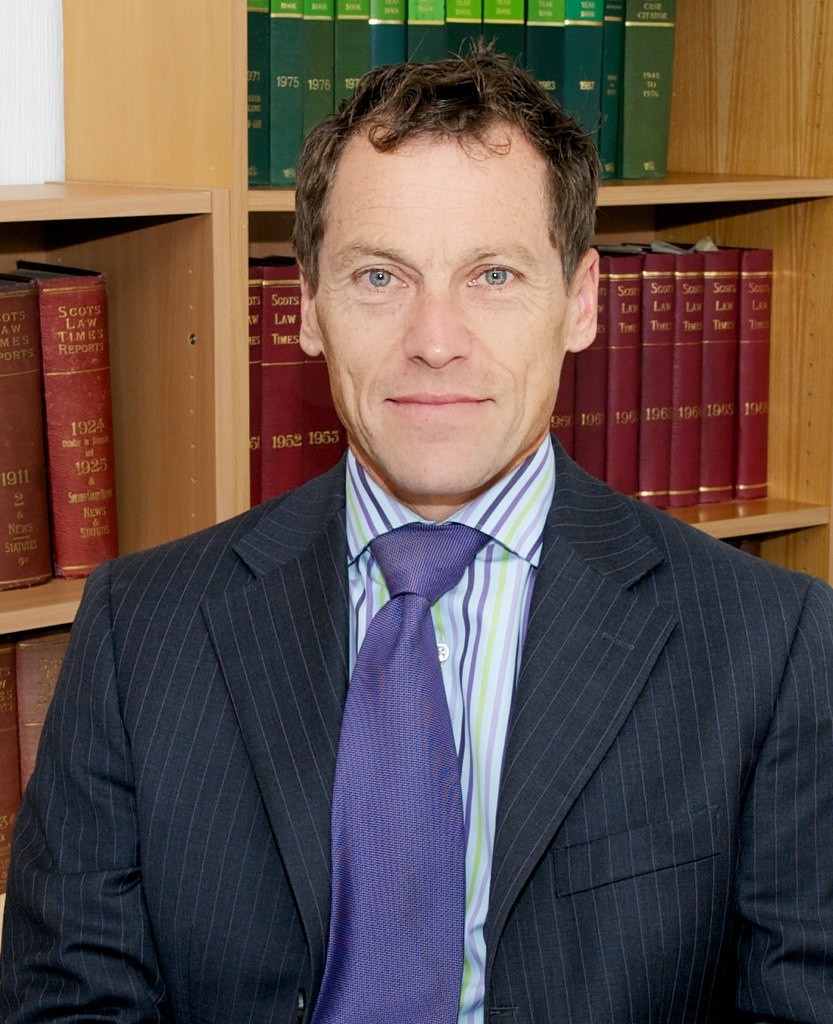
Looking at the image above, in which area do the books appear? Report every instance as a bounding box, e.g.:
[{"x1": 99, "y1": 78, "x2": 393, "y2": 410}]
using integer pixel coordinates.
[
  {"x1": 0, "y1": 630, "x2": 74, "y2": 896},
  {"x1": 0, "y1": 2, "x2": 69, "y2": 181},
  {"x1": 248, "y1": 241, "x2": 775, "y2": 534},
  {"x1": 1, "y1": 254, "x2": 128, "y2": 606},
  {"x1": 248, "y1": 0, "x2": 679, "y2": 186}
]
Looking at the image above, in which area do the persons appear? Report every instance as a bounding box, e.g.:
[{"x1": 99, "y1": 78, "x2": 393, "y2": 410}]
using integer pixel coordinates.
[{"x1": 1, "y1": 39, "x2": 833, "y2": 1024}]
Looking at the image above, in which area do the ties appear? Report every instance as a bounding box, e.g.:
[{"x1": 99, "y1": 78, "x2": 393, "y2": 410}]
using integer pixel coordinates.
[{"x1": 310, "y1": 524, "x2": 493, "y2": 1024}]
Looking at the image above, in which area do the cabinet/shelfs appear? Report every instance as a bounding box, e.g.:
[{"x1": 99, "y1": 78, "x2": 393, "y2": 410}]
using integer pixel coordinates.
[{"x1": 1, "y1": 2, "x2": 833, "y2": 913}]
